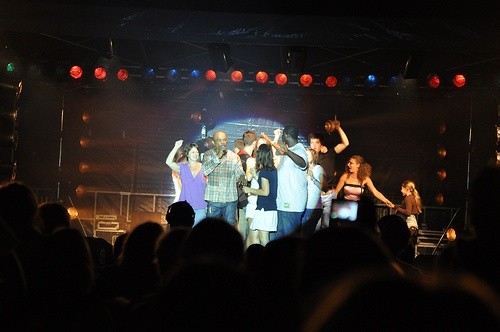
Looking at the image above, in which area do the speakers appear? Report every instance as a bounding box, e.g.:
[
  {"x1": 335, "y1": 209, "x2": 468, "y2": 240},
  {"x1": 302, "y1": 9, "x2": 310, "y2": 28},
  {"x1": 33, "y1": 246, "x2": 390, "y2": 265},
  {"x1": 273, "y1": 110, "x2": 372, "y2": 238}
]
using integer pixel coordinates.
[{"x1": 131, "y1": 211, "x2": 162, "y2": 232}]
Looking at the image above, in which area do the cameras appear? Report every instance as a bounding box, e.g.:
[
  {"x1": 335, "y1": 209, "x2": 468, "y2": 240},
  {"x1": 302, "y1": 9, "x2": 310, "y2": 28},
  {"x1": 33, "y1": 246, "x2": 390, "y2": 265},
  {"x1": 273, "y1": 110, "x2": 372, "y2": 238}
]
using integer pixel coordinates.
[{"x1": 330, "y1": 199, "x2": 359, "y2": 221}]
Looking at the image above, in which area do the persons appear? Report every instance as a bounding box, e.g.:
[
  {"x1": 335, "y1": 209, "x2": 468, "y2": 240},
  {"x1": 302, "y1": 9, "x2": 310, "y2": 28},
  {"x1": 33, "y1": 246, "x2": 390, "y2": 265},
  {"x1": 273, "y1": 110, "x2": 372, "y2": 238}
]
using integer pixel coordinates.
[
  {"x1": 165, "y1": 138, "x2": 208, "y2": 229},
  {"x1": 329, "y1": 154, "x2": 395, "y2": 220},
  {"x1": 201, "y1": 130, "x2": 247, "y2": 231},
  {"x1": 0, "y1": 114, "x2": 500, "y2": 332},
  {"x1": 389, "y1": 180, "x2": 423, "y2": 248}
]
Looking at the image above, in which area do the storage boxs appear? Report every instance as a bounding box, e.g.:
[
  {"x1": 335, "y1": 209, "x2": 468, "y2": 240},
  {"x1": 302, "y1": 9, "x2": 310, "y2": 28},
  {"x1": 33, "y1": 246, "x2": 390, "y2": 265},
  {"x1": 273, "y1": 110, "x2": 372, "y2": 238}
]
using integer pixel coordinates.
[
  {"x1": 94, "y1": 215, "x2": 127, "y2": 242},
  {"x1": 415, "y1": 230, "x2": 447, "y2": 257}
]
